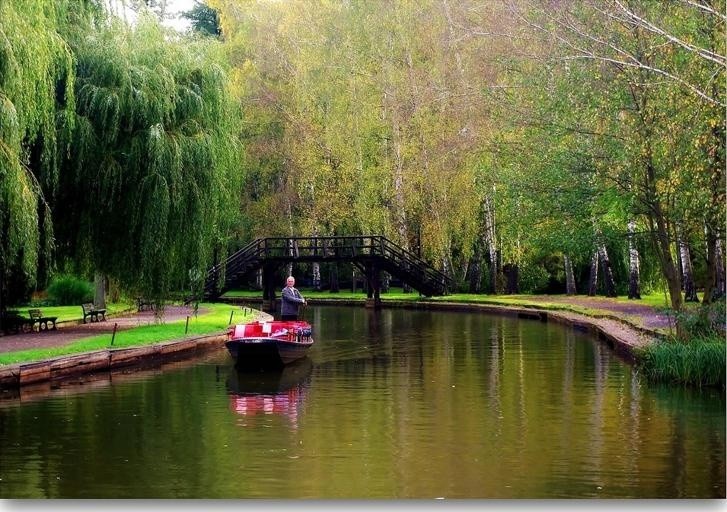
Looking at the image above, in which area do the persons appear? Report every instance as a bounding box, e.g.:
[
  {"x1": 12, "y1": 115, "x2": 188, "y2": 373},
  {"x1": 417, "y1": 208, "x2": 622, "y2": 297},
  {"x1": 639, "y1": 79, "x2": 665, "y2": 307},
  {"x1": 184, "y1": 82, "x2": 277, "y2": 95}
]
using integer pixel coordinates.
[{"x1": 280, "y1": 276, "x2": 308, "y2": 321}]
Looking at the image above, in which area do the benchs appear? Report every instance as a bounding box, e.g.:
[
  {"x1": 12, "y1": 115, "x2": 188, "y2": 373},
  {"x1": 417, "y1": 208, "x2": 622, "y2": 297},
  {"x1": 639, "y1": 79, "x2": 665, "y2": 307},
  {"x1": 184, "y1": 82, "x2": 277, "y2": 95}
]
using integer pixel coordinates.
[
  {"x1": 135, "y1": 295, "x2": 155, "y2": 312},
  {"x1": 82, "y1": 303, "x2": 106, "y2": 323},
  {"x1": 27, "y1": 309, "x2": 57, "y2": 333}
]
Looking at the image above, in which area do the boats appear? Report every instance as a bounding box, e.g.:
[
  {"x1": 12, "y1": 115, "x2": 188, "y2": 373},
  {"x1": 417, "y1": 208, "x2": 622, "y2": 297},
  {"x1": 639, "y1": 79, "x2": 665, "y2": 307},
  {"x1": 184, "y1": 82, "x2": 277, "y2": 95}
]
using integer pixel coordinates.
[
  {"x1": 224, "y1": 336, "x2": 314, "y2": 364},
  {"x1": 225, "y1": 355, "x2": 313, "y2": 395}
]
